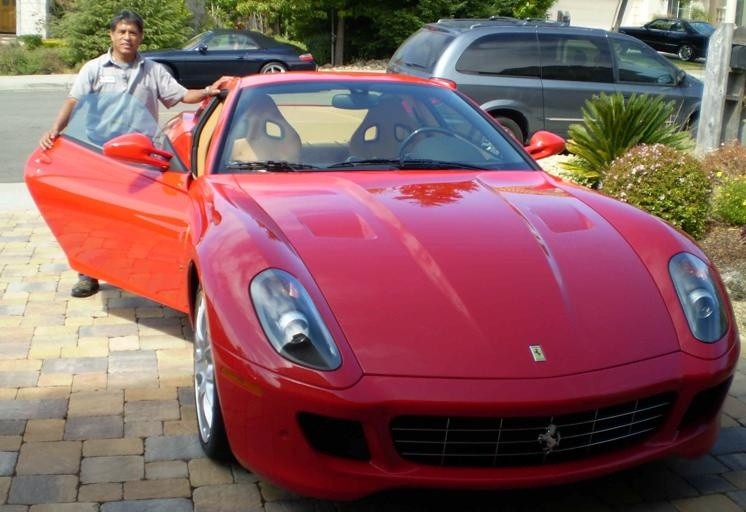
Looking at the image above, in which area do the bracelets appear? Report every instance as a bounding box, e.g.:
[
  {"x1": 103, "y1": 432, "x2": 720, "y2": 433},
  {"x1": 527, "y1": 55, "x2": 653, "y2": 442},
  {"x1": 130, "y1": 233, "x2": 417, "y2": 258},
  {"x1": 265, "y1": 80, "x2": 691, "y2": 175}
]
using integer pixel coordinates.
[{"x1": 205, "y1": 85, "x2": 212, "y2": 96}]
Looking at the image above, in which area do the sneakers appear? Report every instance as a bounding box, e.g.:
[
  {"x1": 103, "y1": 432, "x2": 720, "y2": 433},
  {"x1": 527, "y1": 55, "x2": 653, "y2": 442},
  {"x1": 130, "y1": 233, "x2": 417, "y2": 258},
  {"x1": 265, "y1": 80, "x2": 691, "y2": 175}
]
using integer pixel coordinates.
[{"x1": 72, "y1": 278, "x2": 98, "y2": 297}]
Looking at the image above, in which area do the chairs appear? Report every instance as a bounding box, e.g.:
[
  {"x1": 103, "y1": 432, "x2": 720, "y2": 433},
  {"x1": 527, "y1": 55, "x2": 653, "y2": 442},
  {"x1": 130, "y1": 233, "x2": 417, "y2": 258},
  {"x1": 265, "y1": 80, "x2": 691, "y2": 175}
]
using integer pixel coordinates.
[
  {"x1": 348, "y1": 94, "x2": 426, "y2": 160},
  {"x1": 219, "y1": 93, "x2": 302, "y2": 170}
]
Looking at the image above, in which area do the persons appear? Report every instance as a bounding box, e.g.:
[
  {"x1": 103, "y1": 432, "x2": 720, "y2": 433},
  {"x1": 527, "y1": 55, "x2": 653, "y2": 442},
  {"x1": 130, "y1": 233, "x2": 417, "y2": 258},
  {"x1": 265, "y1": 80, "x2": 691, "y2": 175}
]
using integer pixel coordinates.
[{"x1": 38, "y1": 10, "x2": 235, "y2": 298}]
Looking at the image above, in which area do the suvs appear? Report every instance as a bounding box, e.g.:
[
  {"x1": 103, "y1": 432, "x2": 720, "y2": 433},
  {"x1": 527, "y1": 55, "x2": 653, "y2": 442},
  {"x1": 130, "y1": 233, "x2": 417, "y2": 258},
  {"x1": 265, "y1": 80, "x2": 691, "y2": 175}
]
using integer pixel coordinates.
[{"x1": 381, "y1": 15, "x2": 706, "y2": 155}]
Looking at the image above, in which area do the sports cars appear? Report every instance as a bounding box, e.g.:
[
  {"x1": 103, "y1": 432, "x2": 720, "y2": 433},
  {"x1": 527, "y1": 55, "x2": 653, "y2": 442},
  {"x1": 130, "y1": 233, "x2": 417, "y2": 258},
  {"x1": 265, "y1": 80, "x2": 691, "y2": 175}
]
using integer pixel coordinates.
[{"x1": 17, "y1": 68, "x2": 742, "y2": 506}]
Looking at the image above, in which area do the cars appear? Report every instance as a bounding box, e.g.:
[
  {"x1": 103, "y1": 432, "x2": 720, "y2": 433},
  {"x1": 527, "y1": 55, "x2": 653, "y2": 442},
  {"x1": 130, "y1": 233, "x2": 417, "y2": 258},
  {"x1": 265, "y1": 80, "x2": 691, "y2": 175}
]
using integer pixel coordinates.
[
  {"x1": 611, "y1": 17, "x2": 719, "y2": 64},
  {"x1": 134, "y1": 25, "x2": 325, "y2": 97}
]
